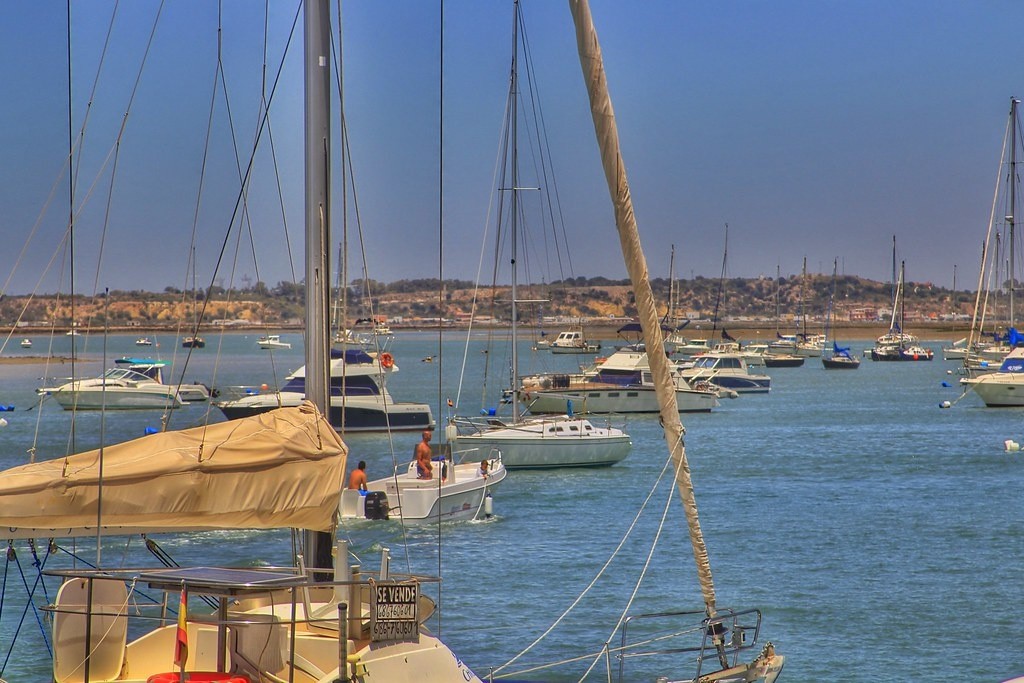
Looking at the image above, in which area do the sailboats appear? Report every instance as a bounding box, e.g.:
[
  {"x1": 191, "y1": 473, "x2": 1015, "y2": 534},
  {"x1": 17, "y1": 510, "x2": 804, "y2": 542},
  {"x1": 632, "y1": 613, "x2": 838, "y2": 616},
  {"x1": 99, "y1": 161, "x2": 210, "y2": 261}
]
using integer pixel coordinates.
[{"x1": 4, "y1": 1, "x2": 1022, "y2": 680}]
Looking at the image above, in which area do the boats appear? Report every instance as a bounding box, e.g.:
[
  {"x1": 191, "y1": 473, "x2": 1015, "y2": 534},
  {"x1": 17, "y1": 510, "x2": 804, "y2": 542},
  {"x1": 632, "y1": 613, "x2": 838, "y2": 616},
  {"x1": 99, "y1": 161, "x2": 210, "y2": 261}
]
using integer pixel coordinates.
[
  {"x1": 183, "y1": 336, "x2": 206, "y2": 349},
  {"x1": 20, "y1": 339, "x2": 32, "y2": 348},
  {"x1": 36, "y1": 368, "x2": 192, "y2": 409},
  {"x1": 136, "y1": 338, "x2": 152, "y2": 346},
  {"x1": 67, "y1": 329, "x2": 80, "y2": 336},
  {"x1": 258, "y1": 335, "x2": 294, "y2": 351}
]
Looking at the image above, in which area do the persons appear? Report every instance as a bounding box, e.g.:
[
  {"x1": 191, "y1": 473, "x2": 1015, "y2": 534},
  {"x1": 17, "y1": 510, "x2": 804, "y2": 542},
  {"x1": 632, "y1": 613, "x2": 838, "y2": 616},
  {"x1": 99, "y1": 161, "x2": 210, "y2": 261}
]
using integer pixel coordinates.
[
  {"x1": 816, "y1": 332, "x2": 820, "y2": 345},
  {"x1": 348, "y1": 461, "x2": 371, "y2": 496},
  {"x1": 476, "y1": 460, "x2": 490, "y2": 480},
  {"x1": 417, "y1": 431, "x2": 433, "y2": 479}
]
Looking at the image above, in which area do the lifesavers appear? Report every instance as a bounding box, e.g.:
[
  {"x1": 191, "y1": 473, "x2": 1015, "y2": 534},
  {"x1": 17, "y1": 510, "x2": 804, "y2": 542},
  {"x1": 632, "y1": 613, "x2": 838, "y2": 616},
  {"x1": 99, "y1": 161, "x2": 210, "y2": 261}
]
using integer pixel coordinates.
[
  {"x1": 380, "y1": 352, "x2": 395, "y2": 369},
  {"x1": 147, "y1": 672, "x2": 253, "y2": 683}
]
